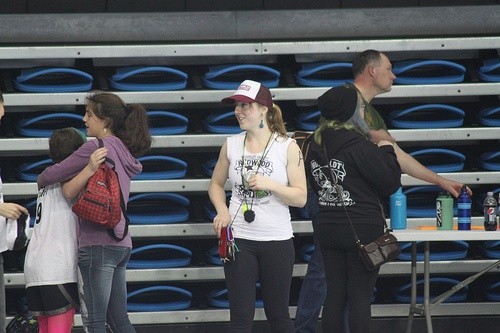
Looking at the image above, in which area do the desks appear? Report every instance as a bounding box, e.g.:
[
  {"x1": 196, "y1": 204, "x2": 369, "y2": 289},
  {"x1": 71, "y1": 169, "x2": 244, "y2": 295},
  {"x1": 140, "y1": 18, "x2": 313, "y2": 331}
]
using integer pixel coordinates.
[{"x1": 384, "y1": 229, "x2": 500, "y2": 333}]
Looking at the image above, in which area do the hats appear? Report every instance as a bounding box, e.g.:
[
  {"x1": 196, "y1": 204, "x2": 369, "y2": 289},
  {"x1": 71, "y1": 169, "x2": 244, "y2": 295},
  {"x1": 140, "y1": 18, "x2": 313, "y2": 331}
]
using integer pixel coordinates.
[
  {"x1": 220, "y1": 80, "x2": 272, "y2": 108},
  {"x1": 317, "y1": 84, "x2": 358, "y2": 123}
]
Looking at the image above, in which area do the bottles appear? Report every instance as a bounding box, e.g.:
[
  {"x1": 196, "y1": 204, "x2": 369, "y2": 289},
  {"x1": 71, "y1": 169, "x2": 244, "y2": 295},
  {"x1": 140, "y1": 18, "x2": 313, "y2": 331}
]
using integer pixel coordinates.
[
  {"x1": 483, "y1": 192, "x2": 497, "y2": 231},
  {"x1": 435, "y1": 191, "x2": 453, "y2": 230}
]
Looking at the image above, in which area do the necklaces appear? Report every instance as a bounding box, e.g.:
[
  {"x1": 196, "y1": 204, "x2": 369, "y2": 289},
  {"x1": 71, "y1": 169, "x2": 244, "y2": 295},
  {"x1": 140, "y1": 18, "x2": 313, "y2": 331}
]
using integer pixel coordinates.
[{"x1": 241, "y1": 128, "x2": 274, "y2": 224}]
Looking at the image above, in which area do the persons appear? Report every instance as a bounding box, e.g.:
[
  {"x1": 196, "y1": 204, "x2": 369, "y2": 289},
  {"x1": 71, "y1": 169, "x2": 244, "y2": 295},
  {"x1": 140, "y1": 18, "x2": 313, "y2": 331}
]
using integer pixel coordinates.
[
  {"x1": 0, "y1": 87, "x2": 152, "y2": 333},
  {"x1": 300, "y1": 85, "x2": 402, "y2": 333},
  {"x1": 208, "y1": 80, "x2": 308, "y2": 333},
  {"x1": 318, "y1": 50, "x2": 474, "y2": 333}
]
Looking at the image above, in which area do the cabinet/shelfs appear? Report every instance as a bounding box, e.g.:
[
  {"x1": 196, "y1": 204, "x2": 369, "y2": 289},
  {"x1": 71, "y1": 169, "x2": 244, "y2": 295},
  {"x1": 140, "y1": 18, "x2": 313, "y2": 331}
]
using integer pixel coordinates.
[{"x1": 0, "y1": 37, "x2": 500, "y2": 333}]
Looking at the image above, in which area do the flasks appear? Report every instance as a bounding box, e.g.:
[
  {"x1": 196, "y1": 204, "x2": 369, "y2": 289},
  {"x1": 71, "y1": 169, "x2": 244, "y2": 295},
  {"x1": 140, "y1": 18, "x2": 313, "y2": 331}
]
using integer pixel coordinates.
[
  {"x1": 457, "y1": 184, "x2": 472, "y2": 231},
  {"x1": 390, "y1": 184, "x2": 407, "y2": 229}
]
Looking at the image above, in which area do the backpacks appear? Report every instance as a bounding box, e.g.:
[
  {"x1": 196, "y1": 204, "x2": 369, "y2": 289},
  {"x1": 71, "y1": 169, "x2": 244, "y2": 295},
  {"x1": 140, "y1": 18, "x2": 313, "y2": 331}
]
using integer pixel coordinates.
[{"x1": 72, "y1": 139, "x2": 131, "y2": 241}]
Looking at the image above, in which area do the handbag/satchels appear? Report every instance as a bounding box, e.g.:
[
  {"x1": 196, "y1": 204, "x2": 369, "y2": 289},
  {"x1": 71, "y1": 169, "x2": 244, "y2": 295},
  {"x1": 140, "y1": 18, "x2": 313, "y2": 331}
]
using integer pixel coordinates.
[
  {"x1": 357, "y1": 232, "x2": 401, "y2": 270},
  {"x1": 218, "y1": 226, "x2": 240, "y2": 263}
]
